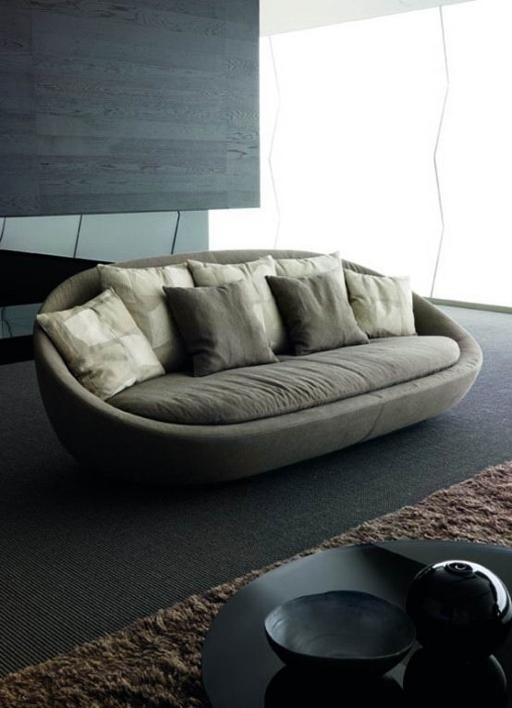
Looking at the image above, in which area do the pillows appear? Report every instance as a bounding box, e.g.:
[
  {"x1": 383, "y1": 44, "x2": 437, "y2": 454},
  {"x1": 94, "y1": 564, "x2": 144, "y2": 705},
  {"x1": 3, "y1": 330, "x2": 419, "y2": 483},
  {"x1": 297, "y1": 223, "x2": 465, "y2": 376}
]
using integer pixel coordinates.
[
  {"x1": 184, "y1": 255, "x2": 291, "y2": 360},
  {"x1": 342, "y1": 270, "x2": 420, "y2": 338},
  {"x1": 161, "y1": 279, "x2": 278, "y2": 379},
  {"x1": 33, "y1": 287, "x2": 165, "y2": 402},
  {"x1": 96, "y1": 262, "x2": 198, "y2": 374},
  {"x1": 263, "y1": 265, "x2": 369, "y2": 356},
  {"x1": 273, "y1": 252, "x2": 346, "y2": 276}
]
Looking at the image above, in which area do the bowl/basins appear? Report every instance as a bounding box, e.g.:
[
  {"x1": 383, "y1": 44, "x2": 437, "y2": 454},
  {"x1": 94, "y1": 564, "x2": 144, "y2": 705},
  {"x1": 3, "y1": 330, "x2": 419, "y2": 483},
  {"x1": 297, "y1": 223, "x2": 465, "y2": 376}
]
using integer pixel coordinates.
[{"x1": 264, "y1": 590, "x2": 415, "y2": 684}]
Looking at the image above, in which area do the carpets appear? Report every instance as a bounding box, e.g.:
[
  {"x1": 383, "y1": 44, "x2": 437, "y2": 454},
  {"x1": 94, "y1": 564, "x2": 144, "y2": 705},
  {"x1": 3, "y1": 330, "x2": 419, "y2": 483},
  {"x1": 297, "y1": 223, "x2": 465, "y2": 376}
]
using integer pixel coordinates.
[{"x1": 0, "y1": 457, "x2": 512, "y2": 708}]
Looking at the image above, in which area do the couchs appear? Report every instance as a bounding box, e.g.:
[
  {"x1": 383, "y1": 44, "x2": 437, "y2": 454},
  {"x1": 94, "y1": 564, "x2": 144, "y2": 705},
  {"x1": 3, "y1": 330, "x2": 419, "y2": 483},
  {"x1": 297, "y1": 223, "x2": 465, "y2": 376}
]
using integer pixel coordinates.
[{"x1": 30, "y1": 247, "x2": 484, "y2": 489}]
